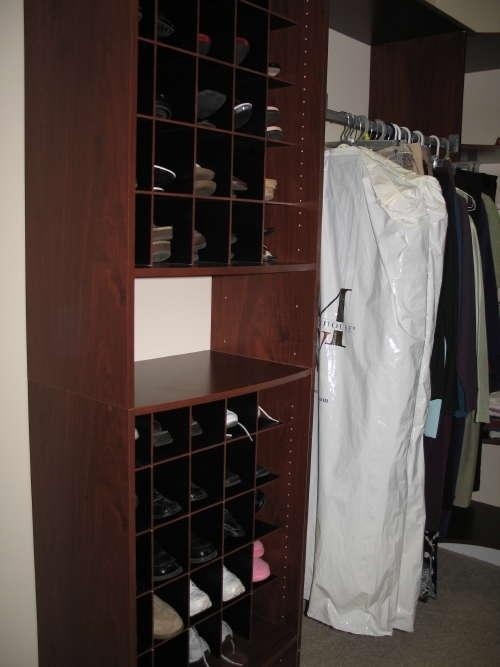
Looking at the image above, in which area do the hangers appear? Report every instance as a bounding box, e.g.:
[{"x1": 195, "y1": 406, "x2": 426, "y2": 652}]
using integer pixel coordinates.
[{"x1": 331, "y1": 111, "x2": 477, "y2": 212}]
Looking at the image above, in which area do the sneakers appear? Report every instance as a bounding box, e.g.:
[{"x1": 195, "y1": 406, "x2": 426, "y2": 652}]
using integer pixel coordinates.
[
  {"x1": 234, "y1": 103, "x2": 252, "y2": 129},
  {"x1": 154, "y1": 595, "x2": 184, "y2": 640},
  {"x1": 269, "y1": 63, "x2": 280, "y2": 77},
  {"x1": 267, "y1": 126, "x2": 283, "y2": 139},
  {"x1": 151, "y1": 241, "x2": 171, "y2": 262},
  {"x1": 222, "y1": 620, "x2": 243, "y2": 666},
  {"x1": 225, "y1": 410, "x2": 254, "y2": 442},
  {"x1": 192, "y1": 230, "x2": 207, "y2": 262},
  {"x1": 150, "y1": 224, "x2": 173, "y2": 241},
  {"x1": 196, "y1": 180, "x2": 216, "y2": 196},
  {"x1": 258, "y1": 404, "x2": 280, "y2": 426},
  {"x1": 153, "y1": 186, "x2": 164, "y2": 192},
  {"x1": 153, "y1": 421, "x2": 173, "y2": 447},
  {"x1": 154, "y1": 164, "x2": 176, "y2": 179},
  {"x1": 195, "y1": 164, "x2": 215, "y2": 180},
  {"x1": 197, "y1": 89, "x2": 227, "y2": 129},
  {"x1": 223, "y1": 567, "x2": 245, "y2": 601},
  {"x1": 266, "y1": 107, "x2": 280, "y2": 126},
  {"x1": 254, "y1": 541, "x2": 264, "y2": 558},
  {"x1": 252, "y1": 559, "x2": 270, "y2": 582},
  {"x1": 156, "y1": 100, "x2": 172, "y2": 120},
  {"x1": 236, "y1": 37, "x2": 248, "y2": 64},
  {"x1": 191, "y1": 421, "x2": 202, "y2": 436},
  {"x1": 190, "y1": 580, "x2": 212, "y2": 616},
  {"x1": 265, "y1": 179, "x2": 278, "y2": 200},
  {"x1": 189, "y1": 626, "x2": 211, "y2": 667},
  {"x1": 158, "y1": 15, "x2": 174, "y2": 37},
  {"x1": 199, "y1": 34, "x2": 211, "y2": 56}
]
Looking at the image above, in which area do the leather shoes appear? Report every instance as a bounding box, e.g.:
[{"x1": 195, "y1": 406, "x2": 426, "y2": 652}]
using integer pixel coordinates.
[
  {"x1": 223, "y1": 518, "x2": 245, "y2": 537},
  {"x1": 255, "y1": 489, "x2": 264, "y2": 513},
  {"x1": 191, "y1": 541, "x2": 217, "y2": 563},
  {"x1": 256, "y1": 465, "x2": 270, "y2": 478},
  {"x1": 153, "y1": 555, "x2": 183, "y2": 581},
  {"x1": 231, "y1": 177, "x2": 248, "y2": 191},
  {"x1": 153, "y1": 491, "x2": 182, "y2": 519},
  {"x1": 190, "y1": 484, "x2": 207, "y2": 501},
  {"x1": 225, "y1": 473, "x2": 241, "y2": 488}
]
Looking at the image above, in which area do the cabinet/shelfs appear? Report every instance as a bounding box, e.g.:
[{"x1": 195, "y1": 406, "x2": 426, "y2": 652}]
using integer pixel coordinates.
[{"x1": 22, "y1": 1, "x2": 331, "y2": 665}]
